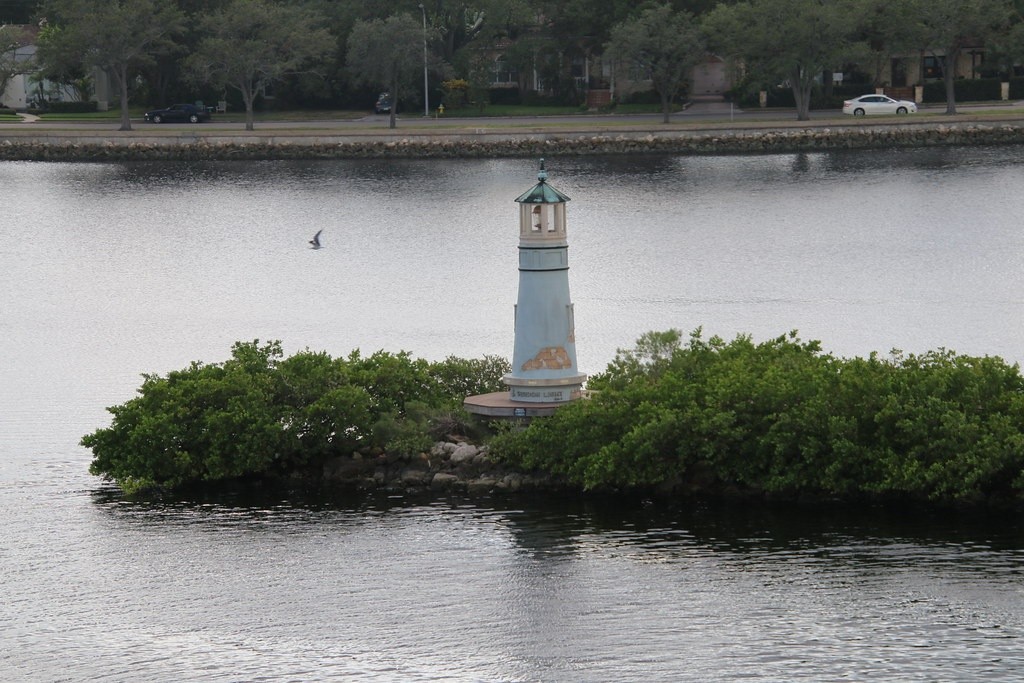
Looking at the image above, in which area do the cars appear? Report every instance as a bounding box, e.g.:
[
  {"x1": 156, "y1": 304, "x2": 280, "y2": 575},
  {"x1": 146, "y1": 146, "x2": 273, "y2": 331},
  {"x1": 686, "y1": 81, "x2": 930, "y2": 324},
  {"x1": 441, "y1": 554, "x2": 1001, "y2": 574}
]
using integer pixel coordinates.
[
  {"x1": 144, "y1": 103, "x2": 211, "y2": 123},
  {"x1": 843, "y1": 94, "x2": 918, "y2": 116}
]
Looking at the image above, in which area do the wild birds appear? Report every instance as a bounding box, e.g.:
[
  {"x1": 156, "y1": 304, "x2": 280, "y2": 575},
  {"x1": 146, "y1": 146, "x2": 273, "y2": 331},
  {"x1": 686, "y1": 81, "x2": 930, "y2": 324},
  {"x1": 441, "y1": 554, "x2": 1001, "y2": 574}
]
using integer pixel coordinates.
[{"x1": 308, "y1": 228, "x2": 324, "y2": 250}]
